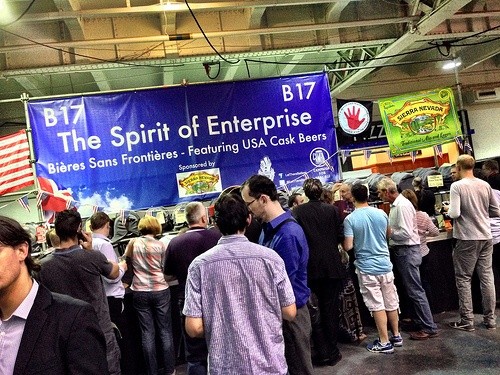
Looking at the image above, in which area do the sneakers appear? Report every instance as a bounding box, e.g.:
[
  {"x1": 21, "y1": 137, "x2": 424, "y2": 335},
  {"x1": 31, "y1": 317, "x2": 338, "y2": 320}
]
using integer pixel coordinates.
[
  {"x1": 366, "y1": 337, "x2": 394, "y2": 353},
  {"x1": 481, "y1": 320, "x2": 497, "y2": 330},
  {"x1": 391, "y1": 333, "x2": 404, "y2": 347},
  {"x1": 449, "y1": 321, "x2": 475, "y2": 331}
]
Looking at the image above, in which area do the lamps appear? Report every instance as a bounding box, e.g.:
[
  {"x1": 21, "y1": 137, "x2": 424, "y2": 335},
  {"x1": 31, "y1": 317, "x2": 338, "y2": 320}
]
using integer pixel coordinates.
[{"x1": 437, "y1": 57, "x2": 462, "y2": 69}]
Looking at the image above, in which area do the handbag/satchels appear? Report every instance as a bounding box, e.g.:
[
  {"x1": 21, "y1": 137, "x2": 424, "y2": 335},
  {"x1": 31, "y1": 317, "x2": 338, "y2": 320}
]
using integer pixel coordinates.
[{"x1": 121, "y1": 239, "x2": 135, "y2": 284}]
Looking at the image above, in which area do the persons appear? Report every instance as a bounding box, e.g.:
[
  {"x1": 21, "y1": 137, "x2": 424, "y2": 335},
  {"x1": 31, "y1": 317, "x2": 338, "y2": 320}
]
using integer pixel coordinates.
[
  {"x1": 164, "y1": 202, "x2": 223, "y2": 375},
  {"x1": 343, "y1": 184, "x2": 403, "y2": 352},
  {"x1": 182, "y1": 195, "x2": 297, "y2": 375},
  {"x1": 293, "y1": 178, "x2": 345, "y2": 366},
  {"x1": 0, "y1": 216, "x2": 107, "y2": 375},
  {"x1": 125, "y1": 216, "x2": 176, "y2": 375},
  {"x1": 377, "y1": 179, "x2": 439, "y2": 340},
  {"x1": 240, "y1": 175, "x2": 314, "y2": 375},
  {"x1": 0, "y1": 160, "x2": 500, "y2": 375},
  {"x1": 443, "y1": 154, "x2": 500, "y2": 331}
]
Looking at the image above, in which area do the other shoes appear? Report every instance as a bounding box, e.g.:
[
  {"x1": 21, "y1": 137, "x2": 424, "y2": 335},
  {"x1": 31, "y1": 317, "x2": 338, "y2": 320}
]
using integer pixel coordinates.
[
  {"x1": 168, "y1": 368, "x2": 178, "y2": 375},
  {"x1": 312, "y1": 349, "x2": 342, "y2": 366},
  {"x1": 352, "y1": 333, "x2": 367, "y2": 346}
]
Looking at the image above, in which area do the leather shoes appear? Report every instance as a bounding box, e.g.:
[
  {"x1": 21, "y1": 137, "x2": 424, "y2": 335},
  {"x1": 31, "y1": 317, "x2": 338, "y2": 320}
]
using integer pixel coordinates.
[
  {"x1": 401, "y1": 318, "x2": 411, "y2": 325},
  {"x1": 410, "y1": 330, "x2": 438, "y2": 340}
]
